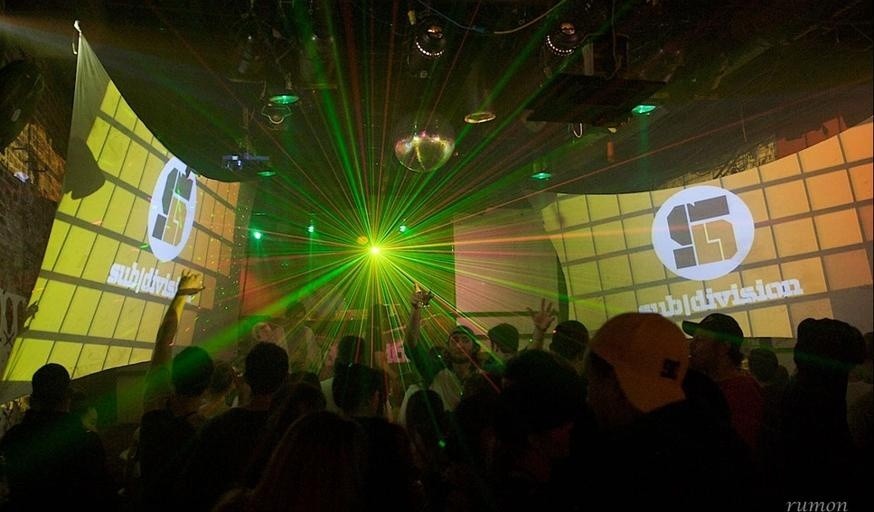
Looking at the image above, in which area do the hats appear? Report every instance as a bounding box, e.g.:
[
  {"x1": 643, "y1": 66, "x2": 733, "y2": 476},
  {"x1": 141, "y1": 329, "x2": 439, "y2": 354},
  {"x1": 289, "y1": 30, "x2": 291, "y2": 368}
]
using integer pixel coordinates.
[
  {"x1": 591, "y1": 309, "x2": 687, "y2": 413},
  {"x1": 681, "y1": 310, "x2": 747, "y2": 352}
]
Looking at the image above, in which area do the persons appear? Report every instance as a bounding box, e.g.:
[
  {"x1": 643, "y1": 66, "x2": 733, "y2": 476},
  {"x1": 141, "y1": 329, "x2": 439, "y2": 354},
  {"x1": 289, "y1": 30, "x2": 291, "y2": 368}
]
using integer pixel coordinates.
[{"x1": 0, "y1": 268, "x2": 874, "y2": 512}]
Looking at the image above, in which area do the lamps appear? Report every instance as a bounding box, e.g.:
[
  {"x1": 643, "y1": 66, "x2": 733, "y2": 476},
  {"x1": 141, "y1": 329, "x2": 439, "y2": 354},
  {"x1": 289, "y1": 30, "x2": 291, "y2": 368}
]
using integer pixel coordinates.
[{"x1": 231, "y1": 5, "x2": 585, "y2": 125}]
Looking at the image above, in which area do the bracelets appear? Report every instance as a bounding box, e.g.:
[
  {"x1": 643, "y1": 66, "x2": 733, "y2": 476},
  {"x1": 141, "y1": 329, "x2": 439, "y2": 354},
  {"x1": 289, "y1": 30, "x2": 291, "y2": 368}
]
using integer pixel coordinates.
[{"x1": 411, "y1": 303, "x2": 419, "y2": 310}]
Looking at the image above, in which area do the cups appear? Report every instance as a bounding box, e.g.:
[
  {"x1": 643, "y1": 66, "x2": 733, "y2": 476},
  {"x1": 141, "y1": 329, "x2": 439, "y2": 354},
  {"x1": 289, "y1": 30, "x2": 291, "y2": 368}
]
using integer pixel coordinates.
[{"x1": 417, "y1": 290, "x2": 430, "y2": 307}]
